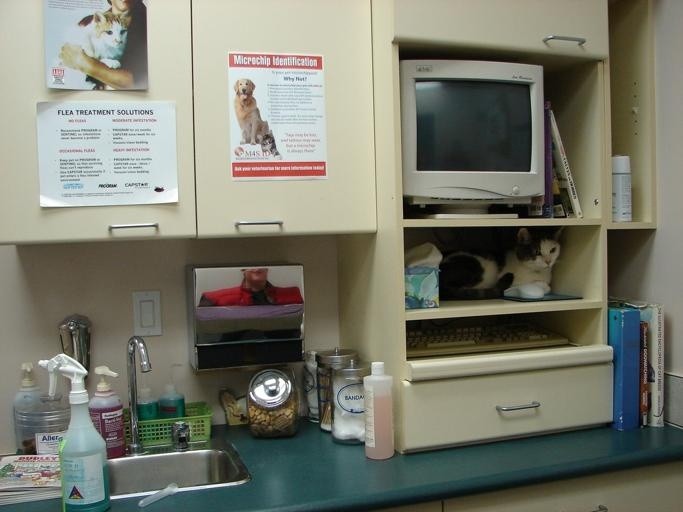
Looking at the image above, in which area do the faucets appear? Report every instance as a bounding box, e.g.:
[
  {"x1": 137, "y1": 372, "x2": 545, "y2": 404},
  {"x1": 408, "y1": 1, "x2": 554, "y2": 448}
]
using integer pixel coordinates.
[{"x1": 125, "y1": 330, "x2": 153, "y2": 453}]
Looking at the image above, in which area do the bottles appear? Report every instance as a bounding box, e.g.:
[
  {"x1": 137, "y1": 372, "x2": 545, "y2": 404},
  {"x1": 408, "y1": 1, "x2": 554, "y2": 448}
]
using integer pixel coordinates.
[
  {"x1": 611, "y1": 154, "x2": 633, "y2": 224},
  {"x1": 136, "y1": 384, "x2": 185, "y2": 420},
  {"x1": 245, "y1": 348, "x2": 394, "y2": 460},
  {"x1": 34, "y1": 393, "x2": 70, "y2": 455}
]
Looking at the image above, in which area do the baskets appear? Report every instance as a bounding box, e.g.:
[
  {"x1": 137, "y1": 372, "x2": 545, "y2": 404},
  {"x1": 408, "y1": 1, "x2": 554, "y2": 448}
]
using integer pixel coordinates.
[{"x1": 124, "y1": 401, "x2": 214, "y2": 446}]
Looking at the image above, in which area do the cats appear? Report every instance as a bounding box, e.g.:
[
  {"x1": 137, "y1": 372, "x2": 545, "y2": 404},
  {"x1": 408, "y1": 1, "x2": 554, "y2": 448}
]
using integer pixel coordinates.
[
  {"x1": 256, "y1": 129, "x2": 282, "y2": 160},
  {"x1": 71, "y1": 8, "x2": 130, "y2": 70},
  {"x1": 439, "y1": 226, "x2": 566, "y2": 301}
]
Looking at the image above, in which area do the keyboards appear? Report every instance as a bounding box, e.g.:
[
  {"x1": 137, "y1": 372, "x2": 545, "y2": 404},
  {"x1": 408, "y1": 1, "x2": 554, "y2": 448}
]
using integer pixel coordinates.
[{"x1": 407, "y1": 319, "x2": 570, "y2": 358}]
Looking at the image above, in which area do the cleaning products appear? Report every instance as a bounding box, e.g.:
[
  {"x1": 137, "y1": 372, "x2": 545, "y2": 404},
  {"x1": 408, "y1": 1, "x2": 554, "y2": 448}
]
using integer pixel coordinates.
[
  {"x1": 37, "y1": 349, "x2": 112, "y2": 511},
  {"x1": 362, "y1": 361, "x2": 396, "y2": 460},
  {"x1": 13, "y1": 361, "x2": 39, "y2": 454}
]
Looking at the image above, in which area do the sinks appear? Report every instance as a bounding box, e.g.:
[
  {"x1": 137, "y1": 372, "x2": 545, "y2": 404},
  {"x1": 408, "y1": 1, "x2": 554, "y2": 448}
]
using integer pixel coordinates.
[{"x1": 108, "y1": 436, "x2": 253, "y2": 500}]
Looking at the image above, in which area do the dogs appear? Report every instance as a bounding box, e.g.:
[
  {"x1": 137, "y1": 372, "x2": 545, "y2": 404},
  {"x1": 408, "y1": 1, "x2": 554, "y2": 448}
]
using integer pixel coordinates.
[{"x1": 233, "y1": 78, "x2": 269, "y2": 146}]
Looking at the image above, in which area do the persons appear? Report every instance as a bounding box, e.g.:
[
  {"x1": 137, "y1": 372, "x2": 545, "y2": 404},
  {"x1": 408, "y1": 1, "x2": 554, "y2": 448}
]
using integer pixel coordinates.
[
  {"x1": 60, "y1": 0, "x2": 148, "y2": 90},
  {"x1": 197, "y1": 267, "x2": 304, "y2": 307}
]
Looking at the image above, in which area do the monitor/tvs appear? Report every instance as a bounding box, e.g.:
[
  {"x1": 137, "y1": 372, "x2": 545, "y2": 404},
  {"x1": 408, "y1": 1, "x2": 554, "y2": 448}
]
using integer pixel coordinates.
[{"x1": 400, "y1": 58, "x2": 546, "y2": 218}]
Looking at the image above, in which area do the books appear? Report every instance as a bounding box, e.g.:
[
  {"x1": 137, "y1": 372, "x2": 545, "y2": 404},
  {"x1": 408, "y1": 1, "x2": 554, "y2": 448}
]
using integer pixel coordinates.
[
  {"x1": 608, "y1": 296, "x2": 665, "y2": 432},
  {"x1": 526, "y1": 100, "x2": 584, "y2": 219},
  {"x1": 0, "y1": 453, "x2": 62, "y2": 506}
]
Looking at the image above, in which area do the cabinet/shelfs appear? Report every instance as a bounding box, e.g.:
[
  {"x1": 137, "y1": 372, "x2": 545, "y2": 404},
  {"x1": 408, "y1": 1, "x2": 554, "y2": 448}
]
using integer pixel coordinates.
[
  {"x1": 1, "y1": 1, "x2": 195, "y2": 245},
  {"x1": 340, "y1": 0, "x2": 616, "y2": 454},
  {"x1": 195, "y1": 2, "x2": 378, "y2": 240}
]
